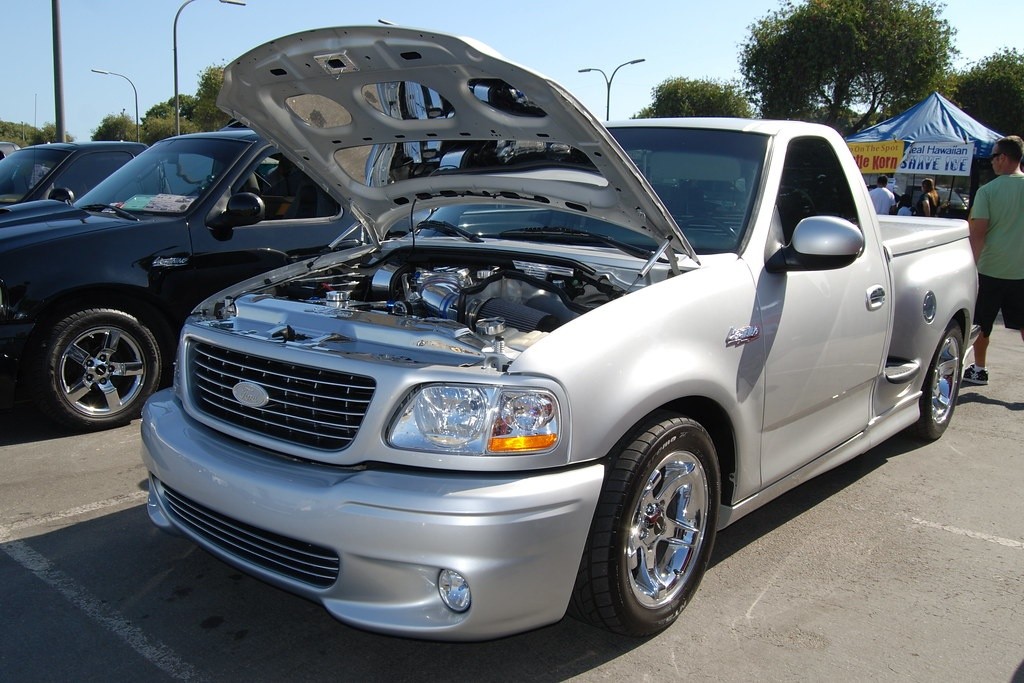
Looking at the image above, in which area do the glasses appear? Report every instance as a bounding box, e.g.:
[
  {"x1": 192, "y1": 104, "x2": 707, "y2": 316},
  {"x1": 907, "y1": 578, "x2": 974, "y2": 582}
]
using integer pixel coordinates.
[{"x1": 989, "y1": 152, "x2": 1006, "y2": 162}]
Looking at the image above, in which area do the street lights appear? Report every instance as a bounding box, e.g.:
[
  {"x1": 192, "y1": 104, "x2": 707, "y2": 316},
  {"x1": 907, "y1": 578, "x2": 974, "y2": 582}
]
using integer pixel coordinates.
[
  {"x1": 91, "y1": 69, "x2": 140, "y2": 141},
  {"x1": 174, "y1": 0, "x2": 246, "y2": 137},
  {"x1": 578, "y1": 58, "x2": 647, "y2": 122}
]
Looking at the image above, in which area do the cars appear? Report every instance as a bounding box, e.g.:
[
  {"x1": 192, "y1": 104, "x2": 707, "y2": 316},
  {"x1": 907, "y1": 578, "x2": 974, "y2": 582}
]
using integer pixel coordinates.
[
  {"x1": 1, "y1": 120, "x2": 432, "y2": 435},
  {"x1": 1, "y1": 141, "x2": 153, "y2": 212}
]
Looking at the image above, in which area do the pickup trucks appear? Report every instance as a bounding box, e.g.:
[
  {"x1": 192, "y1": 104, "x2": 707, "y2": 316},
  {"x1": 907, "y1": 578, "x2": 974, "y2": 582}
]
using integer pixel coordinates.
[{"x1": 139, "y1": 17, "x2": 981, "y2": 644}]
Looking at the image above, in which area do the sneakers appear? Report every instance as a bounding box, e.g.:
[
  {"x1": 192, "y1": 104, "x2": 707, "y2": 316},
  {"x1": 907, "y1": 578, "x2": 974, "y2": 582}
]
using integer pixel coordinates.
[{"x1": 962, "y1": 363, "x2": 989, "y2": 385}]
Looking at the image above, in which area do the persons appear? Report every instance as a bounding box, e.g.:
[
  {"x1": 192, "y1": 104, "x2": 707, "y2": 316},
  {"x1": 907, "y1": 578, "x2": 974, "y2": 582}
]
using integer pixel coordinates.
[
  {"x1": 869, "y1": 173, "x2": 940, "y2": 218},
  {"x1": 963, "y1": 135, "x2": 1024, "y2": 386}
]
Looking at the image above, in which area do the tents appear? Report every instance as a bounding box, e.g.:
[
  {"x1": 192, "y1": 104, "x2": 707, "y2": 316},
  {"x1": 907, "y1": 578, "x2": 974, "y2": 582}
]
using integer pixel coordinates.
[{"x1": 845, "y1": 91, "x2": 1005, "y2": 209}]
